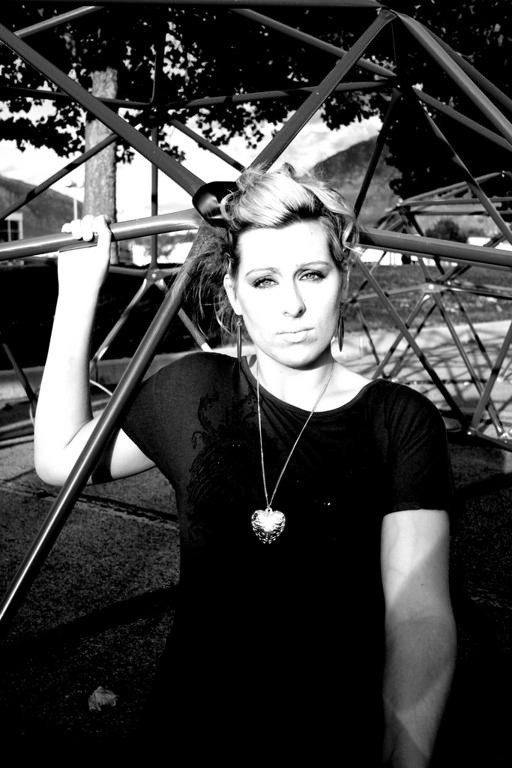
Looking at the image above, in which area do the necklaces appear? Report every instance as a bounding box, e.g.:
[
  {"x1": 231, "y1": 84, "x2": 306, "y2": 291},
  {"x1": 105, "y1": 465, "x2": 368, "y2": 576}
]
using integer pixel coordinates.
[{"x1": 244, "y1": 365, "x2": 341, "y2": 548}]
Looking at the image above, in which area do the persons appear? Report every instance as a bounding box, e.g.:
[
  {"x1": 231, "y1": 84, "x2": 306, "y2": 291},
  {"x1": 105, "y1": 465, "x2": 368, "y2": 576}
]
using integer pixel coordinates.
[{"x1": 34, "y1": 166, "x2": 460, "y2": 766}]
